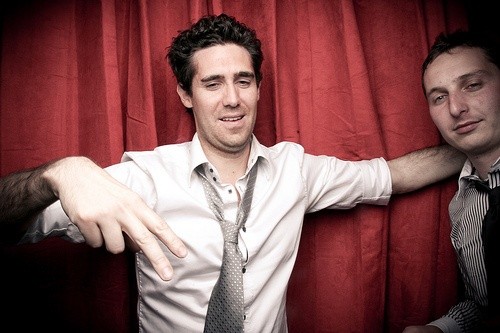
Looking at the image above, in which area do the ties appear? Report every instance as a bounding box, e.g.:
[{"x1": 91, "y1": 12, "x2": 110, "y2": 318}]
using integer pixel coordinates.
[
  {"x1": 473, "y1": 180, "x2": 500, "y2": 333},
  {"x1": 195, "y1": 158, "x2": 258, "y2": 333}
]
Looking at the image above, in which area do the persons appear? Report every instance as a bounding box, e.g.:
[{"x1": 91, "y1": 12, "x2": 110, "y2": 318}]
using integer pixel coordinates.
[
  {"x1": 9, "y1": 14, "x2": 466, "y2": 332},
  {"x1": 403, "y1": 25, "x2": 499, "y2": 332}
]
